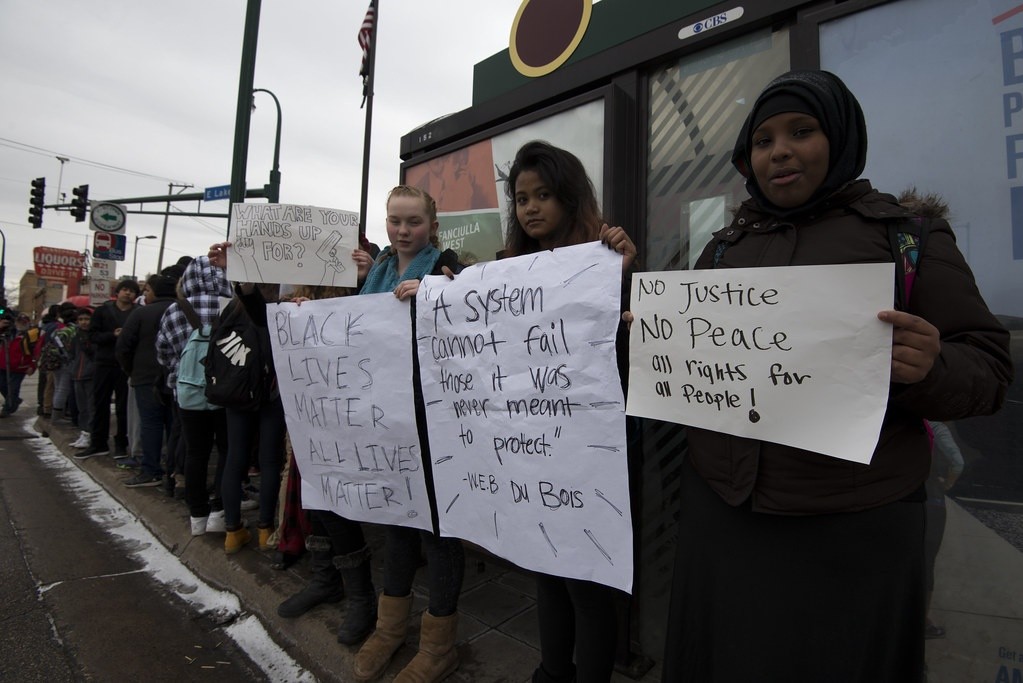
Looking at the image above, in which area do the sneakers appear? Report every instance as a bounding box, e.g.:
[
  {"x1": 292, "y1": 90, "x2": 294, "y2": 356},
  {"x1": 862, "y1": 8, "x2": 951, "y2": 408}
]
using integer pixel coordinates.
[{"x1": 189, "y1": 509, "x2": 248, "y2": 536}]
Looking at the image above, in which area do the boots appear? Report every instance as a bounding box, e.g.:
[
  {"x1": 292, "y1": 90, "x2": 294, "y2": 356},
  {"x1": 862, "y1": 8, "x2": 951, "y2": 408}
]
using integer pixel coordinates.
[
  {"x1": 277, "y1": 534, "x2": 344, "y2": 618},
  {"x1": 352, "y1": 591, "x2": 416, "y2": 682},
  {"x1": 113, "y1": 435, "x2": 129, "y2": 459},
  {"x1": 73, "y1": 434, "x2": 110, "y2": 458},
  {"x1": 390, "y1": 608, "x2": 460, "y2": 683},
  {"x1": 332, "y1": 542, "x2": 378, "y2": 644},
  {"x1": 50, "y1": 409, "x2": 72, "y2": 424},
  {"x1": 69, "y1": 431, "x2": 90, "y2": 448}
]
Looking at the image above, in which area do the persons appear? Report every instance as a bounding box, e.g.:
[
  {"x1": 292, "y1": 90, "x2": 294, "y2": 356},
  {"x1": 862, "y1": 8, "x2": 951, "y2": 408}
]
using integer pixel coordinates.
[
  {"x1": 0, "y1": 233, "x2": 381, "y2": 644},
  {"x1": 925, "y1": 421, "x2": 964, "y2": 639},
  {"x1": 441, "y1": 142, "x2": 639, "y2": 683},
  {"x1": 351, "y1": 186, "x2": 467, "y2": 683},
  {"x1": 622, "y1": 69, "x2": 1012, "y2": 683}
]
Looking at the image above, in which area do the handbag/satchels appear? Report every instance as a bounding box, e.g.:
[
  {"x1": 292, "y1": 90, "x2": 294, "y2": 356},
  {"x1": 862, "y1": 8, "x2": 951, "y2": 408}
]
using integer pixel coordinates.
[
  {"x1": 148, "y1": 365, "x2": 178, "y2": 417},
  {"x1": 200, "y1": 280, "x2": 276, "y2": 409}
]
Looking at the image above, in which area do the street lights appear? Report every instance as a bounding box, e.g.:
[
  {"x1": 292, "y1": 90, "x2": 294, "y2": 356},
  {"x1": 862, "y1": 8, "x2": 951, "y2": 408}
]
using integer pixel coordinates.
[
  {"x1": 250, "y1": 87, "x2": 284, "y2": 202},
  {"x1": 132, "y1": 235, "x2": 156, "y2": 284}
]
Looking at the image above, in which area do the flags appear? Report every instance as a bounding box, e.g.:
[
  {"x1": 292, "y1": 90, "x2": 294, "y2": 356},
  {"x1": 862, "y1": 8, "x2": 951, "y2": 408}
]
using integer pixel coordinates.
[{"x1": 358, "y1": 0, "x2": 375, "y2": 108}]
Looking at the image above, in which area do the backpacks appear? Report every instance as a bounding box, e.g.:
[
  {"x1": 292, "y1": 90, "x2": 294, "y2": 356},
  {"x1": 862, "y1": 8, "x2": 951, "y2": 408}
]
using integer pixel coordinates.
[
  {"x1": 37, "y1": 329, "x2": 61, "y2": 371},
  {"x1": 174, "y1": 297, "x2": 224, "y2": 411}
]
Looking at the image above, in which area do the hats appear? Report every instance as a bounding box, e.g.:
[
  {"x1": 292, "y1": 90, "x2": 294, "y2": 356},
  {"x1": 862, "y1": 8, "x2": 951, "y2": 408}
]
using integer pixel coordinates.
[{"x1": 750, "y1": 90, "x2": 821, "y2": 133}]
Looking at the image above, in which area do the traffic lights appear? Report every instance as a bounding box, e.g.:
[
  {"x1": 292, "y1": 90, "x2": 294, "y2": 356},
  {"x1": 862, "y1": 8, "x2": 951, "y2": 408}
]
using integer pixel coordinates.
[
  {"x1": 28, "y1": 177, "x2": 45, "y2": 229},
  {"x1": 0, "y1": 299, "x2": 7, "y2": 317},
  {"x1": 70, "y1": 185, "x2": 88, "y2": 222}
]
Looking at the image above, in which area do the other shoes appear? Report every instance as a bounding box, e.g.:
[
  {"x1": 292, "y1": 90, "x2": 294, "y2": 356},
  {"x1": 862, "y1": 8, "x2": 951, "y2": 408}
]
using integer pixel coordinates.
[
  {"x1": 240, "y1": 499, "x2": 258, "y2": 510},
  {"x1": 115, "y1": 454, "x2": 142, "y2": 469},
  {"x1": 255, "y1": 519, "x2": 275, "y2": 551},
  {"x1": 122, "y1": 468, "x2": 163, "y2": 487},
  {"x1": 925, "y1": 623, "x2": 946, "y2": 639},
  {"x1": 224, "y1": 521, "x2": 252, "y2": 555},
  {"x1": 160, "y1": 471, "x2": 213, "y2": 499},
  {"x1": 242, "y1": 484, "x2": 260, "y2": 497}
]
